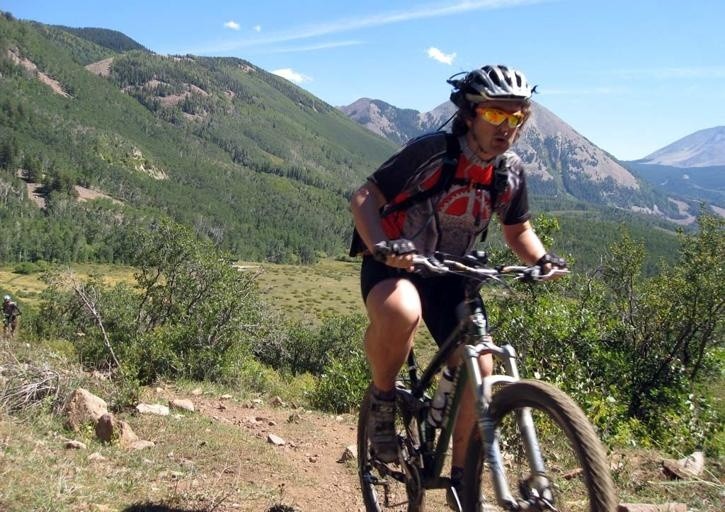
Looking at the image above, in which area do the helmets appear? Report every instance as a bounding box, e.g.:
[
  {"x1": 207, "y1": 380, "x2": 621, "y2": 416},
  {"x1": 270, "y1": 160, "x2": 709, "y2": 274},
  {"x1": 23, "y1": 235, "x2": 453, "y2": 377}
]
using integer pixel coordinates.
[{"x1": 447, "y1": 63, "x2": 533, "y2": 107}]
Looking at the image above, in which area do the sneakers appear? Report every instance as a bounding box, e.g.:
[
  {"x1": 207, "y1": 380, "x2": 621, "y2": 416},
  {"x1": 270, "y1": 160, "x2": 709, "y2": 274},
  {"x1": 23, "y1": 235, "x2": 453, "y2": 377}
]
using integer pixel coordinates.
[
  {"x1": 366, "y1": 380, "x2": 401, "y2": 464},
  {"x1": 446, "y1": 478, "x2": 498, "y2": 511}
]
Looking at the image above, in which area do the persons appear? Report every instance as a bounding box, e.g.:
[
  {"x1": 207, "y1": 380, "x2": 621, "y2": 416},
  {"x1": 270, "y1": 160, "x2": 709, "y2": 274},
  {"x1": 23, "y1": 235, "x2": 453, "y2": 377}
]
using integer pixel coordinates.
[{"x1": 350, "y1": 65, "x2": 567, "y2": 512}]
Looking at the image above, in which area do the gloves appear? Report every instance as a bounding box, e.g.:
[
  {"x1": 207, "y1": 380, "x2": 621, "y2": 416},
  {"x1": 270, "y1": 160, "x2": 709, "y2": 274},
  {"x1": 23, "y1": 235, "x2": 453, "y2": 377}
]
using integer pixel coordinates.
[
  {"x1": 536, "y1": 252, "x2": 568, "y2": 272},
  {"x1": 371, "y1": 239, "x2": 417, "y2": 268}
]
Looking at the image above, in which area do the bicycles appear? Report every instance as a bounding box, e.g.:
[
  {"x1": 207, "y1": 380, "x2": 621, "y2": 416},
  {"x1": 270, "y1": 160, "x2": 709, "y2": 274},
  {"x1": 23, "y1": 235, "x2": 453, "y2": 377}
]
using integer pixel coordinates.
[{"x1": 357, "y1": 243, "x2": 619, "y2": 512}]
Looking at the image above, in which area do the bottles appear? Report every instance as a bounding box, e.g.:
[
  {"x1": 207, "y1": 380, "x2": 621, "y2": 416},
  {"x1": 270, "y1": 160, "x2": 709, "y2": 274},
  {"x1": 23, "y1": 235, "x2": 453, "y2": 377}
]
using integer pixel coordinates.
[{"x1": 424, "y1": 365, "x2": 458, "y2": 428}]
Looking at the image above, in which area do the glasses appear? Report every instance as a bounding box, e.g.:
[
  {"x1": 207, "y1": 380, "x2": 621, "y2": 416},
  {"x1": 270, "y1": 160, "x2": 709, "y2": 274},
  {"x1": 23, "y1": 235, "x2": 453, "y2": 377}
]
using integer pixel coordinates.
[{"x1": 472, "y1": 105, "x2": 527, "y2": 129}]
